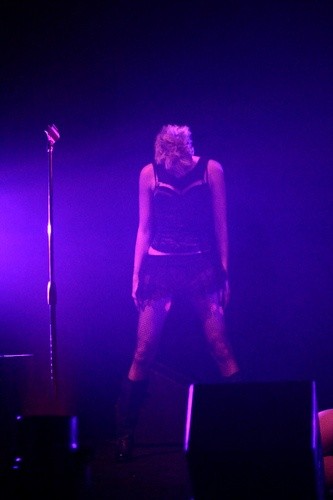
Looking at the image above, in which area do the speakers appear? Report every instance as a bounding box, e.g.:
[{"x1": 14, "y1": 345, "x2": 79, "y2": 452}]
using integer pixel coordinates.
[
  {"x1": 184, "y1": 380, "x2": 326, "y2": 499},
  {"x1": 13, "y1": 413, "x2": 78, "y2": 473}
]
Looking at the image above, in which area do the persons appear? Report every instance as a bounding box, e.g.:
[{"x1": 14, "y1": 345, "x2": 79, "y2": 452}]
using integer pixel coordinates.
[{"x1": 113, "y1": 125, "x2": 240, "y2": 461}]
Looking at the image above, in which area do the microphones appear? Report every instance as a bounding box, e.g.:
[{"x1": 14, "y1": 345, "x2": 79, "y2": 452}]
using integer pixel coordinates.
[{"x1": 44, "y1": 124, "x2": 60, "y2": 152}]
